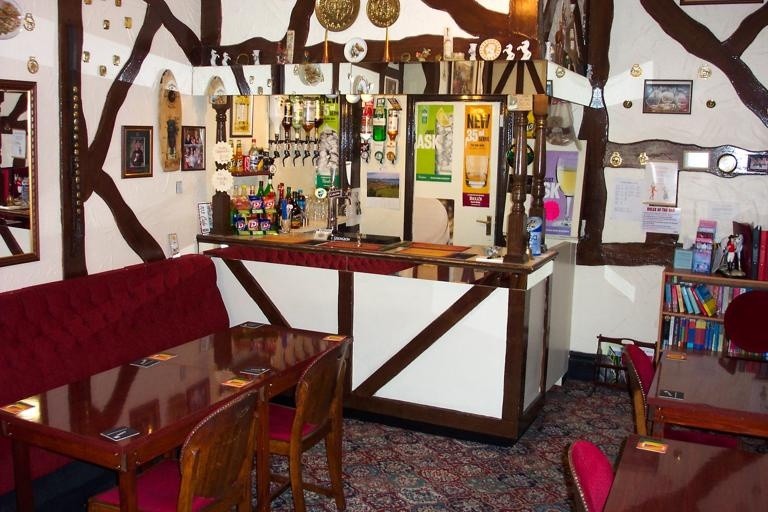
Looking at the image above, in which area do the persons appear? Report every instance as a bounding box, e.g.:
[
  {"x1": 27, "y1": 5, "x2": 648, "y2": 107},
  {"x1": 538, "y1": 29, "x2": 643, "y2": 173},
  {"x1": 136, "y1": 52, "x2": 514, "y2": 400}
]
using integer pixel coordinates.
[
  {"x1": 183, "y1": 129, "x2": 203, "y2": 169},
  {"x1": 131, "y1": 143, "x2": 143, "y2": 166}
]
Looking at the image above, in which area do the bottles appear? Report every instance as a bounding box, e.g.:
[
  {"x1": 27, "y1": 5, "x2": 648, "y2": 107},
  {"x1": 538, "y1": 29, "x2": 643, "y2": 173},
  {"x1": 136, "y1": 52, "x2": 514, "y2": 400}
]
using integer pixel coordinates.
[
  {"x1": 276, "y1": 185, "x2": 285, "y2": 227},
  {"x1": 250, "y1": 185, "x2": 256, "y2": 195},
  {"x1": 264, "y1": 152, "x2": 269, "y2": 170},
  {"x1": 235, "y1": 140, "x2": 244, "y2": 173},
  {"x1": 291, "y1": 191, "x2": 301, "y2": 229},
  {"x1": 227, "y1": 140, "x2": 236, "y2": 172},
  {"x1": 281, "y1": 199, "x2": 289, "y2": 232},
  {"x1": 298, "y1": 189, "x2": 305, "y2": 210},
  {"x1": 256, "y1": 181, "x2": 264, "y2": 197},
  {"x1": 280, "y1": 182, "x2": 284, "y2": 199},
  {"x1": 285, "y1": 187, "x2": 292, "y2": 203},
  {"x1": 249, "y1": 139, "x2": 258, "y2": 172},
  {"x1": 270, "y1": 95, "x2": 398, "y2": 146},
  {"x1": 265, "y1": 179, "x2": 274, "y2": 196},
  {"x1": 241, "y1": 185, "x2": 247, "y2": 196},
  {"x1": 233, "y1": 186, "x2": 239, "y2": 198}
]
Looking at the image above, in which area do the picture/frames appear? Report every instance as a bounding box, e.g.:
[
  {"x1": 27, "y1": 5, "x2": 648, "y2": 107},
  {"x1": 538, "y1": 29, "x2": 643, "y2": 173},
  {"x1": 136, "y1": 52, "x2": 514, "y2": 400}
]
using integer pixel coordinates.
[
  {"x1": 121, "y1": 125, "x2": 153, "y2": 179},
  {"x1": 643, "y1": 79, "x2": 693, "y2": 114},
  {"x1": 230, "y1": 96, "x2": 254, "y2": 138},
  {"x1": 181, "y1": 125, "x2": 206, "y2": 171},
  {"x1": 0, "y1": 79, "x2": 38, "y2": 267}
]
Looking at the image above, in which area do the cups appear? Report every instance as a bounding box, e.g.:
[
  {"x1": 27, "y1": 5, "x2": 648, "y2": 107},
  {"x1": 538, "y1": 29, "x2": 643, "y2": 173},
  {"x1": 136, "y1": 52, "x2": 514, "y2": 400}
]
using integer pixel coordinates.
[
  {"x1": 435, "y1": 120, "x2": 452, "y2": 176},
  {"x1": 467, "y1": 156, "x2": 488, "y2": 188}
]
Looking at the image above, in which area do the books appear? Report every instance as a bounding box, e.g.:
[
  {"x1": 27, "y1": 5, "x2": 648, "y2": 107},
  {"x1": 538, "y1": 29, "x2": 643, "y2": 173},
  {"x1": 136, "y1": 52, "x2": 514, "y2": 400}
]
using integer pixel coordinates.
[{"x1": 661, "y1": 218, "x2": 768, "y2": 360}]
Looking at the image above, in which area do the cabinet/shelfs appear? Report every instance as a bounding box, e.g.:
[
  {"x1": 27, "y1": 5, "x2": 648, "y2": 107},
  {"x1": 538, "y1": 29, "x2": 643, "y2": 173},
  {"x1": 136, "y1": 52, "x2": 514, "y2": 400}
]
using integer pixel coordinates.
[{"x1": 654, "y1": 268, "x2": 768, "y2": 366}]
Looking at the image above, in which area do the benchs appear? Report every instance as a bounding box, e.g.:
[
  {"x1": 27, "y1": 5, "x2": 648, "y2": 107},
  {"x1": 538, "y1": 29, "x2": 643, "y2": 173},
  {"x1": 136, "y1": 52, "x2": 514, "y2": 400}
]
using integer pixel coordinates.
[{"x1": 0, "y1": 254, "x2": 230, "y2": 497}]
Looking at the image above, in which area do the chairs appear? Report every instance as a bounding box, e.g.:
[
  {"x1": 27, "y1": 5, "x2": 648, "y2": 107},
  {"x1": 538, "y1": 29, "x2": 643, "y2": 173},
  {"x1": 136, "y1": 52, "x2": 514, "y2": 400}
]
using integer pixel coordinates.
[
  {"x1": 722, "y1": 291, "x2": 768, "y2": 361},
  {"x1": 563, "y1": 344, "x2": 656, "y2": 512},
  {"x1": 267, "y1": 336, "x2": 354, "y2": 512},
  {"x1": 89, "y1": 389, "x2": 258, "y2": 512},
  {"x1": 401, "y1": 198, "x2": 449, "y2": 244}
]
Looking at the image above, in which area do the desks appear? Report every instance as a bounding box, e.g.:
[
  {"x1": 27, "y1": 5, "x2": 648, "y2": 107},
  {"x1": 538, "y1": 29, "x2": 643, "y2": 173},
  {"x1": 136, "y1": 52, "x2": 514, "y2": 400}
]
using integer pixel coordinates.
[
  {"x1": 646, "y1": 345, "x2": 768, "y2": 440},
  {"x1": 603, "y1": 435, "x2": 767, "y2": 511},
  {"x1": 0, "y1": 321, "x2": 354, "y2": 512}
]
